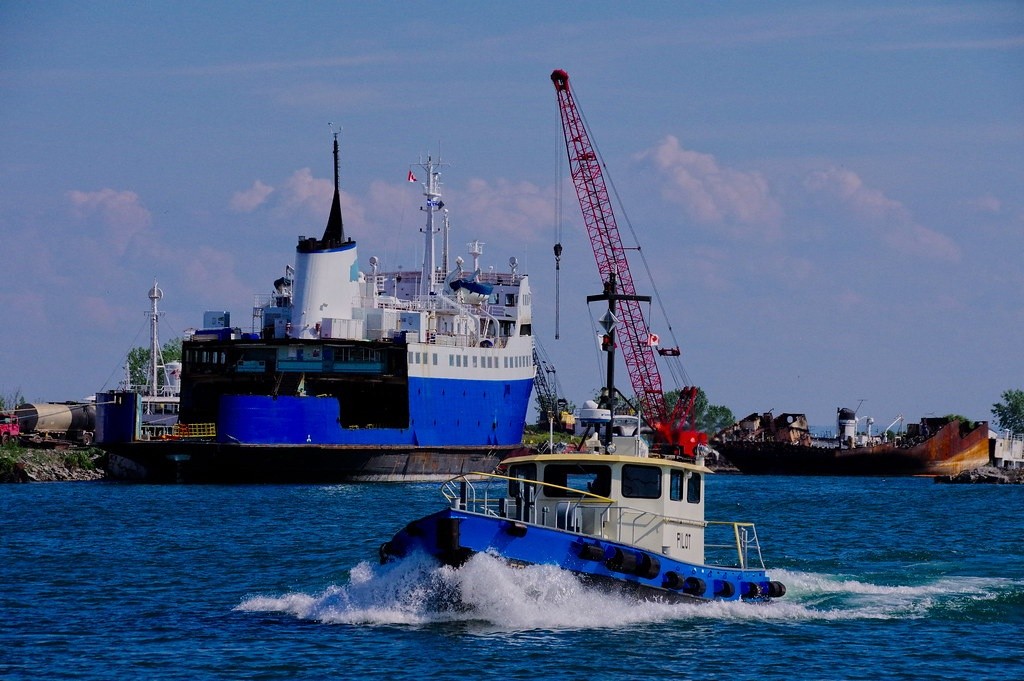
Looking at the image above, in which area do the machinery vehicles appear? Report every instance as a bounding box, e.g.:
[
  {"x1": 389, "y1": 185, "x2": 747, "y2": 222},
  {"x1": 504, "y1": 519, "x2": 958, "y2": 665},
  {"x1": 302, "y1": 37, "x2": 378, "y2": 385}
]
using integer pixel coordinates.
[{"x1": 547, "y1": 68, "x2": 722, "y2": 469}]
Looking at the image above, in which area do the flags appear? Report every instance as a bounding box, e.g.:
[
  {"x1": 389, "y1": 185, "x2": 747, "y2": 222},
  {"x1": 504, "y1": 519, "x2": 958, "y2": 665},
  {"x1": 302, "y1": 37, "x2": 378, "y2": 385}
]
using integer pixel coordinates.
[
  {"x1": 648, "y1": 334, "x2": 660, "y2": 346},
  {"x1": 407, "y1": 170, "x2": 417, "y2": 182},
  {"x1": 427, "y1": 199, "x2": 438, "y2": 206}
]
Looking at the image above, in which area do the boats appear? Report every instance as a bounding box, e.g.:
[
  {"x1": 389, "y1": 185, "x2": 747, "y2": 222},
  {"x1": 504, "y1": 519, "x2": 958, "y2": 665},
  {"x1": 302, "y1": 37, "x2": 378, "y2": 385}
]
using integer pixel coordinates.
[
  {"x1": 561, "y1": 268, "x2": 653, "y2": 457},
  {"x1": 87, "y1": 118, "x2": 540, "y2": 484},
  {"x1": 710, "y1": 405, "x2": 989, "y2": 479},
  {"x1": 378, "y1": 451, "x2": 788, "y2": 604}
]
